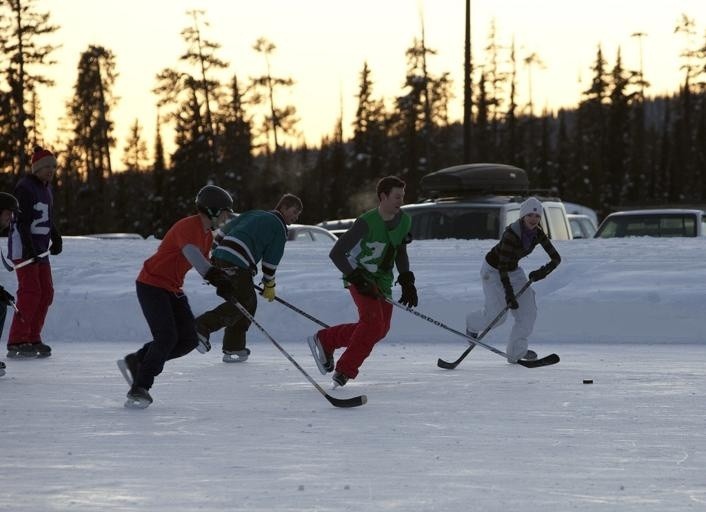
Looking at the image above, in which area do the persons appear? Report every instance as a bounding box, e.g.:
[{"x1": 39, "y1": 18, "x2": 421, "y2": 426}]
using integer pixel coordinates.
[
  {"x1": 6, "y1": 144, "x2": 63, "y2": 353},
  {"x1": 125, "y1": 183, "x2": 238, "y2": 403},
  {"x1": 313, "y1": 175, "x2": 420, "y2": 388},
  {"x1": 194, "y1": 191, "x2": 304, "y2": 358},
  {"x1": 0, "y1": 192, "x2": 23, "y2": 369},
  {"x1": 466, "y1": 194, "x2": 562, "y2": 363}
]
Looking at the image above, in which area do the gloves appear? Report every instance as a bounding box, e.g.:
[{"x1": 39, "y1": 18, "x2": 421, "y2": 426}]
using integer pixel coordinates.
[
  {"x1": 348, "y1": 267, "x2": 378, "y2": 297},
  {"x1": 395, "y1": 271, "x2": 418, "y2": 308},
  {"x1": 261, "y1": 278, "x2": 276, "y2": 301},
  {"x1": 49, "y1": 229, "x2": 62, "y2": 254},
  {"x1": 529, "y1": 270, "x2": 545, "y2": 281},
  {"x1": 505, "y1": 294, "x2": 518, "y2": 310}
]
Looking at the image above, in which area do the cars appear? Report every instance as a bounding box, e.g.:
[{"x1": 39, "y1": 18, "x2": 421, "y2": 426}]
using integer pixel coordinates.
[{"x1": 591, "y1": 209, "x2": 706, "y2": 238}]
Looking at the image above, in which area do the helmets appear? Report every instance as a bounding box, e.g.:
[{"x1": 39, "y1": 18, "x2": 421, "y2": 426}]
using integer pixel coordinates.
[
  {"x1": 195, "y1": 185, "x2": 234, "y2": 213},
  {"x1": 0, "y1": 192, "x2": 22, "y2": 213}
]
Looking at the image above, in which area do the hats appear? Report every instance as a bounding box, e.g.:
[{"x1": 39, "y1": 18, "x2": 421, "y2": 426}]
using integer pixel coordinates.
[
  {"x1": 32, "y1": 147, "x2": 56, "y2": 173},
  {"x1": 520, "y1": 198, "x2": 542, "y2": 217}
]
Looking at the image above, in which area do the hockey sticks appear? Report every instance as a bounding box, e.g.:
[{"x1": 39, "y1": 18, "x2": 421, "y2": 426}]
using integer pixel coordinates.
[
  {"x1": 438, "y1": 282, "x2": 529, "y2": 370},
  {"x1": 7, "y1": 300, "x2": 25, "y2": 321},
  {"x1": 1, "y1": 251, "x2": 51, "y2": 272},
  {"x1": 230, "y1": 296, "x2": 368, "y2": 408},
  {"x1": 382, "y1": 294, "x2": 561, "y2": 368}
]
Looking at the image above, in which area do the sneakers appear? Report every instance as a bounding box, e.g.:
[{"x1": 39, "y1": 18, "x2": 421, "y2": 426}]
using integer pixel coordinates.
[
  {"x1": 125, "y1": 353, "x2": 153, "y2": 403},
  {"x1": 7, "y1": 341, "x2": 52, "y2": 356},
  {"x1": 314, "y1": 333, "x2": 348, "y2": 386},
  {"x1": 223, "y1": 348, "x2": 250, "y2": 354},
  {"x1": 194, "y1": 318, "x2": 211, "y2": 350},
  {"x1": 508, "y1": 350, "x2": 537, "y2": 362}
]
